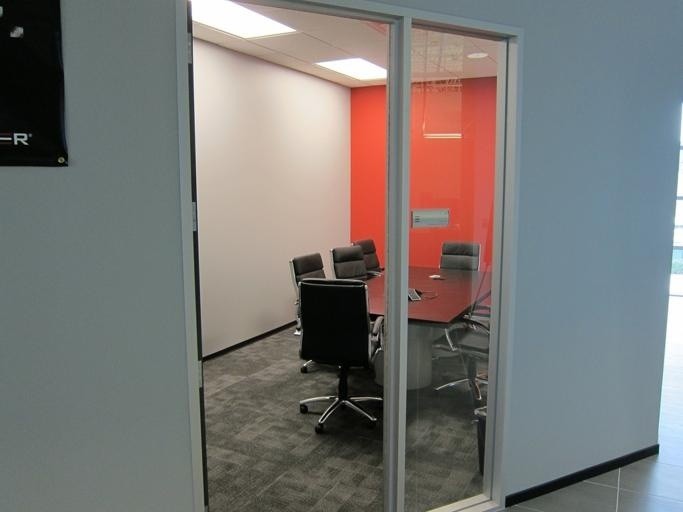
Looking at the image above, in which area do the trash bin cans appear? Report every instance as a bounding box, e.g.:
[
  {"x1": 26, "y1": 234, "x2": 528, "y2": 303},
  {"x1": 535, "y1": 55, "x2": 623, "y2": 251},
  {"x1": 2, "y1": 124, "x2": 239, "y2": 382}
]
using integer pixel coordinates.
[{"x1": 473, "y1": 405, "x2": 488, "y2": 476}]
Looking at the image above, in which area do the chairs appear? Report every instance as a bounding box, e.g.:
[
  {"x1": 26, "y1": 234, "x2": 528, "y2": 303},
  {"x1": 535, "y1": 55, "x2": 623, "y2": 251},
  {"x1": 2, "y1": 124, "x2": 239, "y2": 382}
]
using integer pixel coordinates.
[
  {"x1": 289, "y1": 238, "x2": 383, "y2": 372},
  {"x1": 438, "y1": 240, "x2": 480, "y2": 351},
  {"x1": 298, "y1": 278, "x2": 381, "y2": 432},
  {"x1": 430, "y1": 314, "x2": 492, "y2": 405}
]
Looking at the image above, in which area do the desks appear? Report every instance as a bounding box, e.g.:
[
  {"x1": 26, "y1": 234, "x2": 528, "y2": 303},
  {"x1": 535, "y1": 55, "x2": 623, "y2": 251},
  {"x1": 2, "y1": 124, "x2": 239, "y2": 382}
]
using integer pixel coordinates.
[{"x1": 359, "y1": 265, "x2": 490, "y2": 330}]
